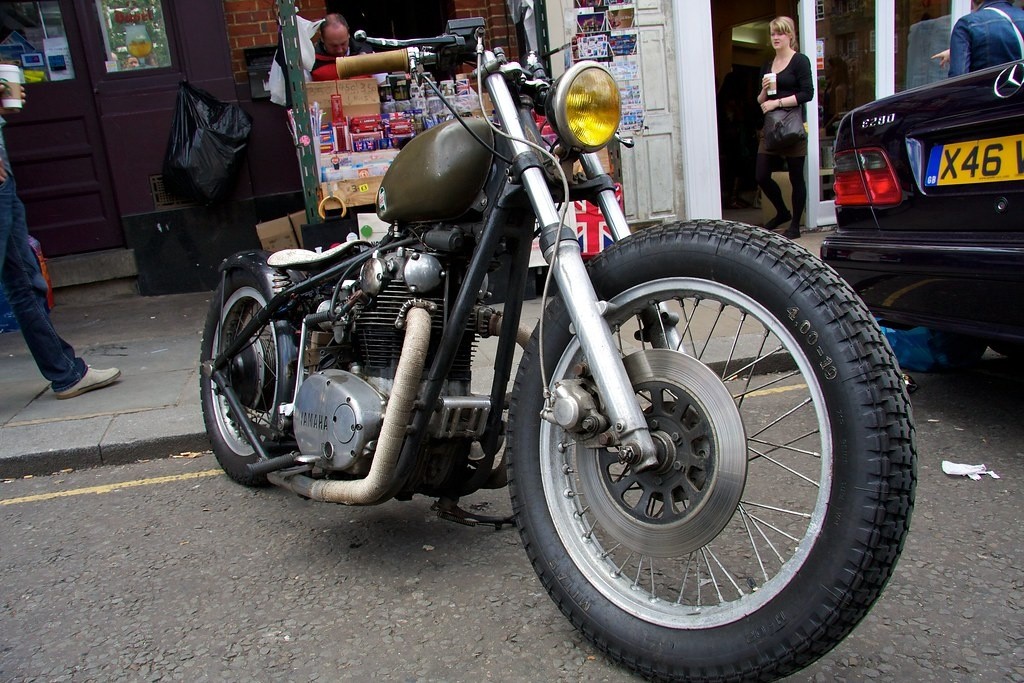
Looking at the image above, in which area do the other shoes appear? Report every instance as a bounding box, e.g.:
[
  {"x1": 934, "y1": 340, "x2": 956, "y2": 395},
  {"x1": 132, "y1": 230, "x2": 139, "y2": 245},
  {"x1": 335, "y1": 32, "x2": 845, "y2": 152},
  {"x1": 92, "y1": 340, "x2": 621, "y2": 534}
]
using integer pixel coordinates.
[
  {"x1": 765, "y1": 210, "x2": 792, "y2": 231},
  {"x1": 781, "y1": 227, "x2": 801, "y2": 238},
  {"x1": 55, "y1": 365, "x2": 122, "y2": 400}
]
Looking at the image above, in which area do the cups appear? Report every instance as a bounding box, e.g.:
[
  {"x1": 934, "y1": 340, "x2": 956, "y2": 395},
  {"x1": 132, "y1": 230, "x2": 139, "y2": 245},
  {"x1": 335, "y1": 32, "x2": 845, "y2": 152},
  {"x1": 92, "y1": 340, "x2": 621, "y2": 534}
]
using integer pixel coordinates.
[
  {"x1": 456, "y1": 80, "x2": 470, "y2": 96},
  {"x1": 0, "y1": 64, "x2": 23, "y2": 108},
  {"x1": 425, "y1": 81, "x2": 437, "y2": 97},
  {"x1": 410, "y1": 83, "x2": 425, "y2": 99},
  {"x1": 440, "y1": 80, "x2": 455, "y2": 96},
  {"x1": 764, "y1": 73, "x2": 776, "y2": 95}
]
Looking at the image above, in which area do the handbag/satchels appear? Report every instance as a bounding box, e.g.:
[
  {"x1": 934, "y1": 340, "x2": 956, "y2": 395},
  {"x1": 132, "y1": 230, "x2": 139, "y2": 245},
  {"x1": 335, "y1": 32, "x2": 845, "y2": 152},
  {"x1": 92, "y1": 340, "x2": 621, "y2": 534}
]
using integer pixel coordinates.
[
  {"x1": 165, "y1": 81, "x2": 259, "y2": 207},
  {"x1": 763, "y1": 104, "x2": 806, "y2": 153},
  {"x1": 300, "y1": 195, "x2": 359, "y2": 253}
]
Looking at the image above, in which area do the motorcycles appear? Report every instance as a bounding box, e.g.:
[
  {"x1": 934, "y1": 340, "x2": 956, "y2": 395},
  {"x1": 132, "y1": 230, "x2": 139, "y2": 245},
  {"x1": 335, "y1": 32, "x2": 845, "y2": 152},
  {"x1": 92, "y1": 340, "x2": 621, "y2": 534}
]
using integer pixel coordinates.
[{"x1": 198, "y1": 7, "x2": 921, "y2": 683}]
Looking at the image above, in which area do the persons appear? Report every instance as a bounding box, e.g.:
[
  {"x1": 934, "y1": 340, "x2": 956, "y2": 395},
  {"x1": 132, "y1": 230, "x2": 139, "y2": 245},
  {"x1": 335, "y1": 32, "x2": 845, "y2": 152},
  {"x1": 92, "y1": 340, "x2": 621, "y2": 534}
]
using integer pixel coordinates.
[
  {"x1": 823, "y1": 57, "x2": 848, "y2": 136},
  {"x1": 755, "y1": 16, "x2": 814, "y2": 239},
  {"x1": 931, "y1": 0, "x2": 1024, "y2": 77},
  {"x1": 0, "y1": 60, "x2": 121, "y2": 400},
  {"x1": 312, "y1": 13, "x2": 389, "y2": 85}
]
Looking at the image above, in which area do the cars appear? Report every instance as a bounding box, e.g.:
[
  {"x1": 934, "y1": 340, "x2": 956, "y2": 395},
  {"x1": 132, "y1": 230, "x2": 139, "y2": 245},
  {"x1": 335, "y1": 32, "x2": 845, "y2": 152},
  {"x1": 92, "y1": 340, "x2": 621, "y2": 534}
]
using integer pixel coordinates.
[{"x1": 816, "y1": 49, "x2": 1024, "y2": 397}]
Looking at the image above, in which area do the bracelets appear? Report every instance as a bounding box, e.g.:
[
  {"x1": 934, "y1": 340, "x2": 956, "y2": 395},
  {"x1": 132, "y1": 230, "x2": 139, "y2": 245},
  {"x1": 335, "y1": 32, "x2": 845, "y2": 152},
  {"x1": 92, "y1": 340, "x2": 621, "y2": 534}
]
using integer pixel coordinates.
[{"x1": 779, "y1": 99, "x2": 783, "y2": 107}]
